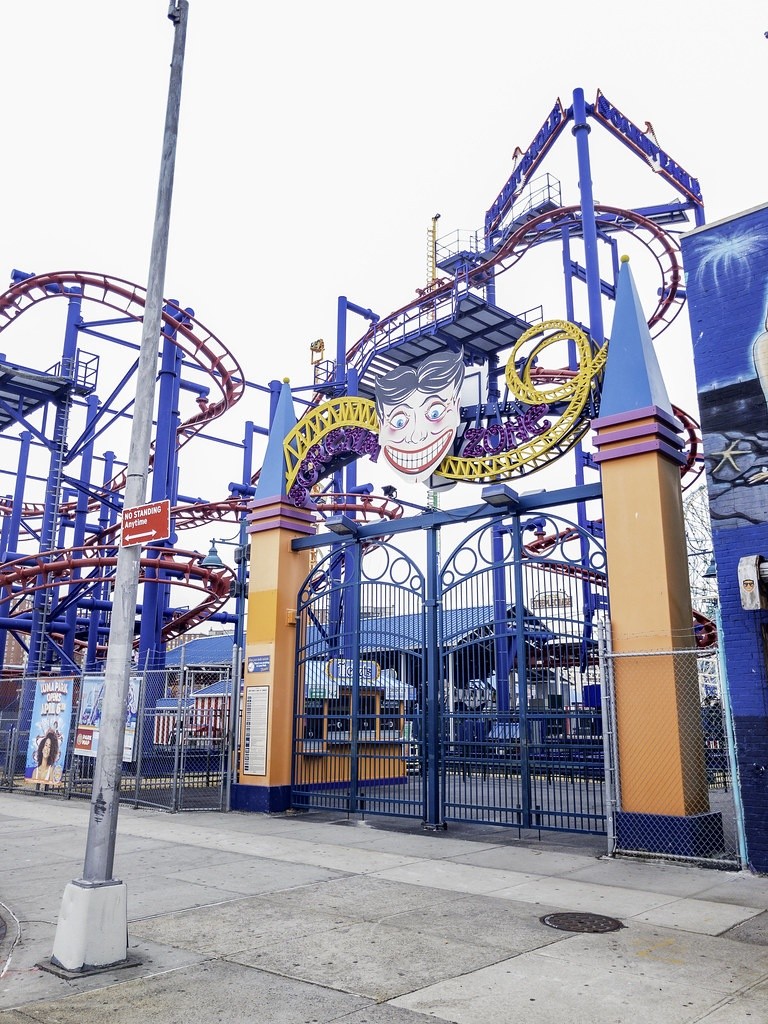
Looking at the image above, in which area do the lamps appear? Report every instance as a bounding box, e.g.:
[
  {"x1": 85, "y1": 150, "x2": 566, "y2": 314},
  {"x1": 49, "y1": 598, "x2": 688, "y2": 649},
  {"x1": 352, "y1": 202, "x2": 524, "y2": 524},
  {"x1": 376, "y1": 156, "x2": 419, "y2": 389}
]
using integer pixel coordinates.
[{"x1": 481, "y1": 484, "x2": 519, "y2": 508}]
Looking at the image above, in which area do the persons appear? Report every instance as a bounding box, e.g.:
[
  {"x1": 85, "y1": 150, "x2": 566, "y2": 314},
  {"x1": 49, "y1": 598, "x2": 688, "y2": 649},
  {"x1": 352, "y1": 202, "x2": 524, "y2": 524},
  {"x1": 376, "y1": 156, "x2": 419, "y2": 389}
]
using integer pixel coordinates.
[{"x1": 31, "y1": 732, "x2": 58, "y2": 780}]
